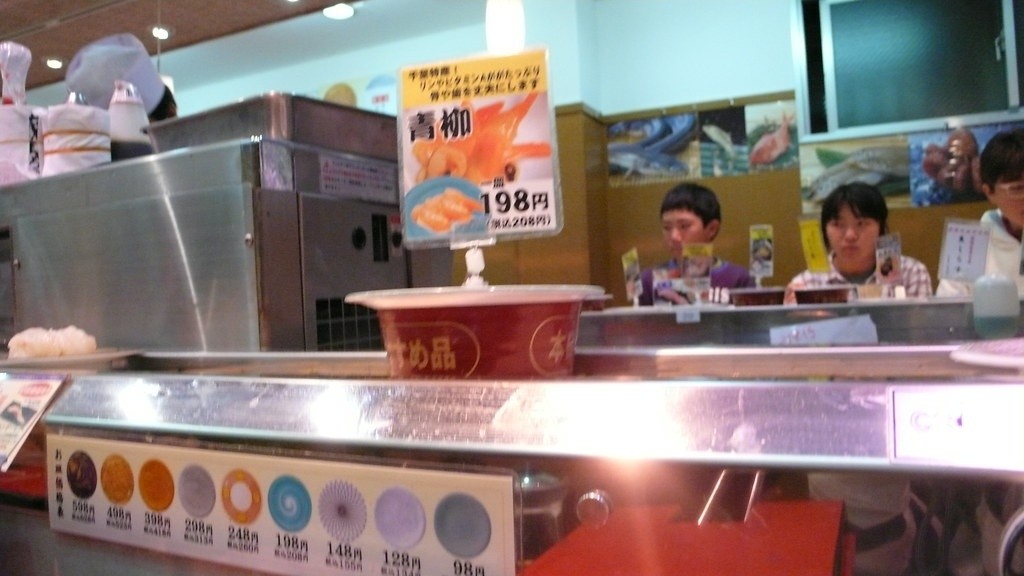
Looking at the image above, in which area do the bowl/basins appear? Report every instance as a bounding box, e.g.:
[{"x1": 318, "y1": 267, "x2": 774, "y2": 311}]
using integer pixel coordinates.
[
  {"x1": 729, "y1": 287, "x2": 785, "y2": 307},
  {"x1": 791, "y1": 288, "x2": 853, "y2": 304}
]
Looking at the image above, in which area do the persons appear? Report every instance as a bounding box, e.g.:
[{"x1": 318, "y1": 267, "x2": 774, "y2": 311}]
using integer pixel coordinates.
[
  {"x1": 7, "y1": 404, "x2": 36, "y2": 424},
  {"x1": 65, "y1": 33, "x2": 176, "y2": 122},
  {"x1": 783, "y1": 181, "x2": 932, "y2": 305},
  {"x1": 934, "y1": 126, "x2": 1024, "y2": 302},
  {"x1": 640, "y1": 182, "x2": 756, "y2": 305}
]
